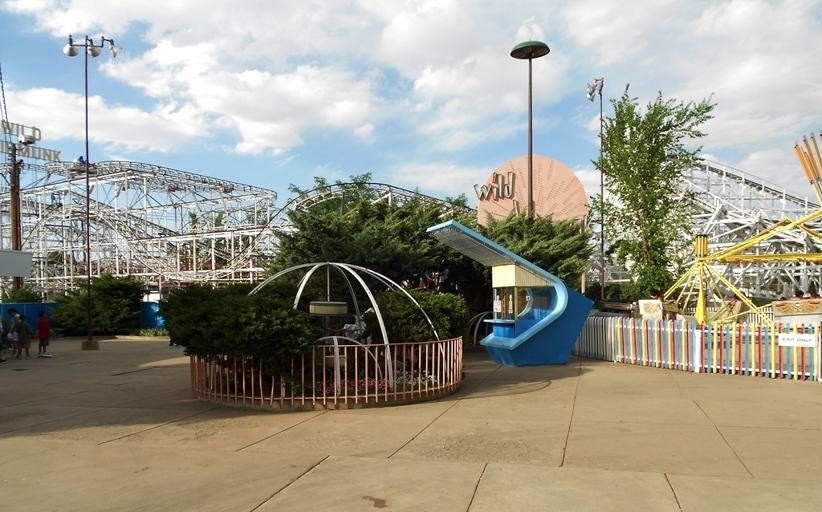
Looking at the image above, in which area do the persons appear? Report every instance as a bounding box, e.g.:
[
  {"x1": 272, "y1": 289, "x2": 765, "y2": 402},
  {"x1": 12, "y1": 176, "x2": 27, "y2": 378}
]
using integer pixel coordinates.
[
  {"x1": 0, "y1": 308, "x2": 33, "y2": 363},
  {"x1": 328, "y1": 316, "x2": 360, "y2": 340},
  {"x1": 37, "y1": 310, "x2": 51, "y2": 355}
]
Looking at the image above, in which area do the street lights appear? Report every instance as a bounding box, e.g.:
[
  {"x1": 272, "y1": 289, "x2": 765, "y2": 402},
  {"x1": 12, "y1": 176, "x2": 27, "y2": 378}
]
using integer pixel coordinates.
[
  {"x1": 62, "y1": 31, "x2": 122, "y2": 352},
  {"x1": 584, "y1": 74, "x2": 606, "y2": 311},
  {"x1": 508, "y1": 38, "x2": 552, "y2": 233}
]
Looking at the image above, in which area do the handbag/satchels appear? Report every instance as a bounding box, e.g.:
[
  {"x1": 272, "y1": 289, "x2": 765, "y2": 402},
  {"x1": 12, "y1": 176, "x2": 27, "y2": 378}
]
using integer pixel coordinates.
[{"x1": 7, "y1": 331, "x2": 19, "y2": 342}]
